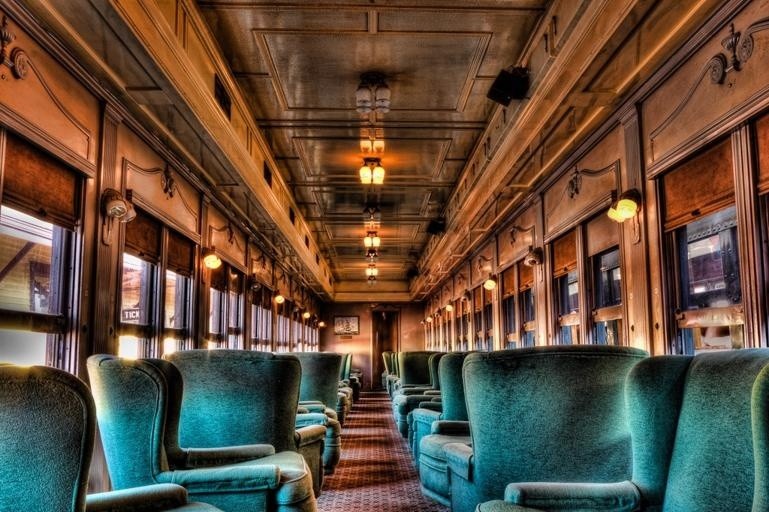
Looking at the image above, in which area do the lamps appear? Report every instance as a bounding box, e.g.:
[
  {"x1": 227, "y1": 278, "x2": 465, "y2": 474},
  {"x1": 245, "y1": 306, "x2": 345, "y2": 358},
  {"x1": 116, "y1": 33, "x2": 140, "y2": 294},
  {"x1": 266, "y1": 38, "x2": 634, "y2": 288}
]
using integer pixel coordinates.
[
  {"x1": 98, "y1": 187, "x2": 137, "y2": 226},
  {"x1": 356, "y1": 76, "x2": 393, "y2": 116}
]
